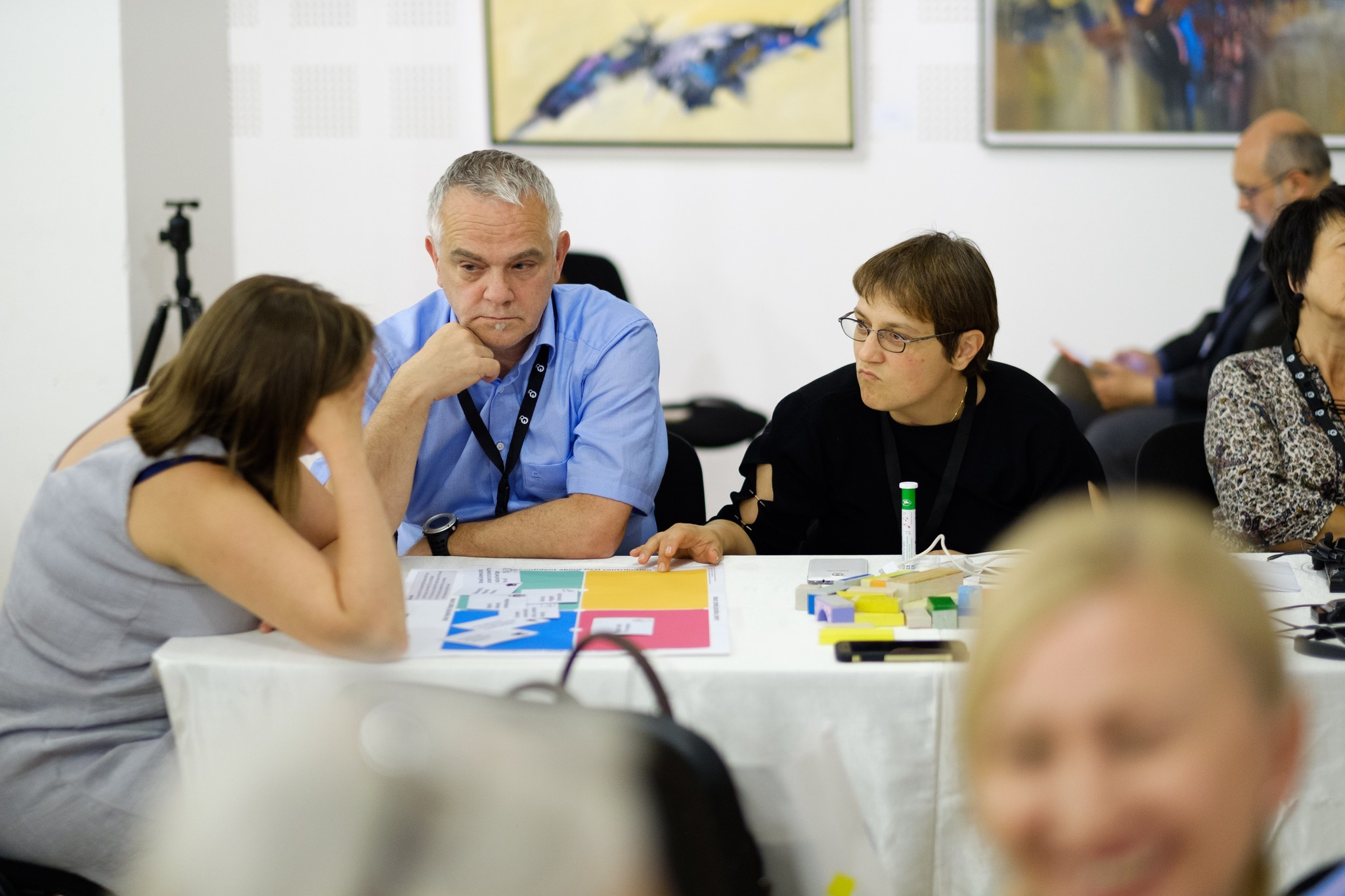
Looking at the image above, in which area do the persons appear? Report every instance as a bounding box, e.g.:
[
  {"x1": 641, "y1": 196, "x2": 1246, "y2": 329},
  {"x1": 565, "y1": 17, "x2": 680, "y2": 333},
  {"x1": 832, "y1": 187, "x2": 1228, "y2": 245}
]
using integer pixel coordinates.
[
  {"x1": 1086, "y1": 110, "x2": 1345, "y2": 506},
  {"x1": 1203, "y1": 185, "x2": 1345, "y2": 553},
  {"x1": 953, "y1": 493, "x2": 1304, "y2": 896},
  {"x1": 630, "y1": 232, "x2": 1108, "y2": 572},
  {"x1": 0, "y1": 274, "x2": 409, "y2": 896},
  {"x1": 312, "y1": 150, "x2": 668, "y2": 558}
]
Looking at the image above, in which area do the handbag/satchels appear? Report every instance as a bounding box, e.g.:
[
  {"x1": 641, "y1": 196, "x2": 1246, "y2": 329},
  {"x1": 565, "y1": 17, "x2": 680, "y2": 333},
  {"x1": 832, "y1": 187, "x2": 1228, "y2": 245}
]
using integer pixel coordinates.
[{"x1": 504, "y1": 632, "x2": 772, "y2": 896}]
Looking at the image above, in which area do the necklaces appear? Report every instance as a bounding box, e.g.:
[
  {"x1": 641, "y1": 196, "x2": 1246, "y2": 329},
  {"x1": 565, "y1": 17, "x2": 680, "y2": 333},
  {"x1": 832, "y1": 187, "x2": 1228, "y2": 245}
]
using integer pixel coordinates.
[{"x1": 950, "y1": 384, "x2": 968, "y2": 422}]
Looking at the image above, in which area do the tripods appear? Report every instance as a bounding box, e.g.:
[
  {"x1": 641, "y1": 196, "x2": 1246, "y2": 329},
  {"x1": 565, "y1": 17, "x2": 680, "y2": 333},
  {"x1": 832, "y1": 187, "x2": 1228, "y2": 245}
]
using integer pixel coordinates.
[{"x1": 128, "y1": 201, "x2": 204, "y2": 396}]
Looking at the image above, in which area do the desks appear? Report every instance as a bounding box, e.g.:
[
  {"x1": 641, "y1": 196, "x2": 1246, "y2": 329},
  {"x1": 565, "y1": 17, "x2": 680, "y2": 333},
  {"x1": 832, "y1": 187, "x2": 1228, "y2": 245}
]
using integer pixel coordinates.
[{"x1": 153, "y1": 552, "x2": 1345, "y2": 896}]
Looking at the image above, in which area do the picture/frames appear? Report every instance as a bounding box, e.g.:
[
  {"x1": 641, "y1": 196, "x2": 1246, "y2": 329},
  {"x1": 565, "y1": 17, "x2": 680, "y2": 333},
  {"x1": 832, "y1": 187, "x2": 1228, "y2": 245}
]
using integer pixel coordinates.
[
  {"x1": 482, "y1": 0, "x2": 871, "y2": 165},
  {"x1": 980, "y1": 0, "x2": 1344, "y2": 152}
]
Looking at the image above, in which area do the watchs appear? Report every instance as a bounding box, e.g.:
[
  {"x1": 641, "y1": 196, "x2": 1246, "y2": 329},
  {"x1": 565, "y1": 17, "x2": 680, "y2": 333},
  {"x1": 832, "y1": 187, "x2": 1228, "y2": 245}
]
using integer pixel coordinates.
[{"x1": 422, "y1": 513, "x2": 459, "y2": 557}]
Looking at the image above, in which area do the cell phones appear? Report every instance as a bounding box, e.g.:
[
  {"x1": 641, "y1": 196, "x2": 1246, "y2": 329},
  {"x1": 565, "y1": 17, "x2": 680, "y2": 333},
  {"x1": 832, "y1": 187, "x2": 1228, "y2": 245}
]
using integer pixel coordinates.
[
  {"x1": 835, "y1": 640, "x2": 969, "y2": 663},
  {"x1": 807, "y1": 558, "x2": 868, "y2": 585}
]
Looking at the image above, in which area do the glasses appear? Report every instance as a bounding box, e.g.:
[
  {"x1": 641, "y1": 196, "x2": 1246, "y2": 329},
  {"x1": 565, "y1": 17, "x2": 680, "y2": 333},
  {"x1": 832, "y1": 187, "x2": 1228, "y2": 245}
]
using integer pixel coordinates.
[
  {"x1": 839, "y1": 311, "x2": 958, "y2": 353},
  {"x1": 1234, "y1": 168, "x2": 1309, "y2": 199}
]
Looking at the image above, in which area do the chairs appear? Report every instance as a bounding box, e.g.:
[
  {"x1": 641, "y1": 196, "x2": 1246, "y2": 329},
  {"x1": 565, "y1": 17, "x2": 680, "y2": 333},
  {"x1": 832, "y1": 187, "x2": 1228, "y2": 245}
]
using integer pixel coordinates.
[
  {"x1": 560, "y1": 254, "x2": 628, "y2": 304},
  {"x1": 587, "y1": 705, "x2": 769, "y2": 896},
  {"x1": 653, "y1": 433, "x2": 707, "y2": 532},
  {"x1": 1137, "y1": 422, "x2": 1221, "y2": 514}
]
